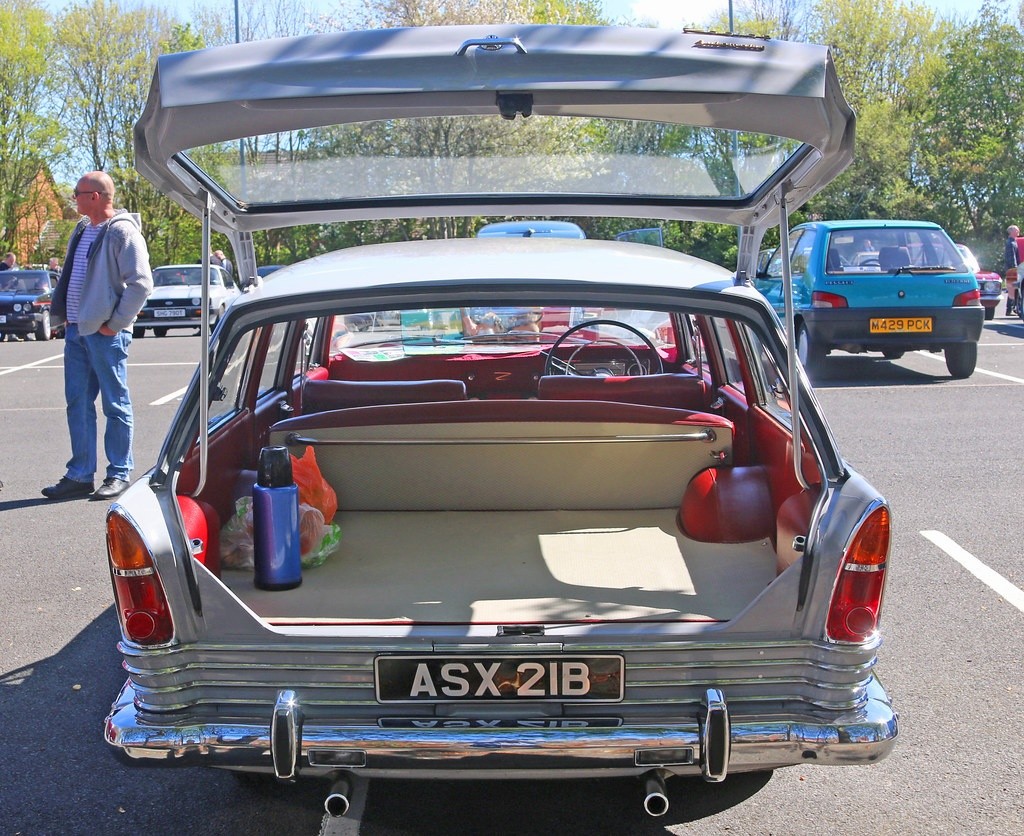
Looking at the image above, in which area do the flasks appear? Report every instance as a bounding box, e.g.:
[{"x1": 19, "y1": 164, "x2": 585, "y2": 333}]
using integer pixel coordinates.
[{"x1": 253, "y1": 444, "x2": 303, "y2": 592}]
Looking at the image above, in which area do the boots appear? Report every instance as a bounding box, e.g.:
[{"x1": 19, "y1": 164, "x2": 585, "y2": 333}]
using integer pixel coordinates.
[{"x1": 1006, "y1": 297, "x2": 1018, "y2": 316}]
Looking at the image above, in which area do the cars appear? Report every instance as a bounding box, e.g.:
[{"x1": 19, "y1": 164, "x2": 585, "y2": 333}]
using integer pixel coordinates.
[
  {"x1": 130, "y1": 264, "x2": 241, "y2": 339},
  {"x1": 0, "y1": 270, "x2": 66, "y2": 341},
  {"x1": 345, "y1": 314, "x2": 379, "y2": 331},
  {"x1": 908, "y1": 244, "x2": 1002, "y2": 321},
  {"x1": 1005, "y1": 237, "x2": 1024, "y2": 320},
  {"x1": 758, "y1": 247, "x2": 850, "y2": 275},
  {"x1": 100, "y1": 25, "x2": 899, "y2": 820},
  {"x1": 256, "y1": 264, "x2": 284, "y2": 279},
  {"x1": 470, "y1": 220, "x2": 588, "y2": 328},
  {"x1": 852, "y1": 251, "x2": 880, "y2": 267},
  {"x1": 752, "y1": 220, "x2": 984, "y2": 380}
]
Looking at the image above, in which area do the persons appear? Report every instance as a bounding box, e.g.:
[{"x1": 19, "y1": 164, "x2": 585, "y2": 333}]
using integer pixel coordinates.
[
  {"x1": 36, "y1": 169, "x2": 156, "y2": 505},
  {"x1": 0, "y1": 245, "x2": 234, "y2": 344},
  {"x1": 1002, "y1": 223, "x2": 1020, "y2": 316}
]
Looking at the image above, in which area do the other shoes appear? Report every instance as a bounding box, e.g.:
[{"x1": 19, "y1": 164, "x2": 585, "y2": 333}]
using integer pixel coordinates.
[{"x1": 8, "y1": 337, "x2": 24, "y2": 342}]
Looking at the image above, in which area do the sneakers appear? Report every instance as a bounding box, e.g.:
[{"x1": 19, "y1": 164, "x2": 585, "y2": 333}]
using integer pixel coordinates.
[
  {"x1": 93, "y1": 477, "x2": 130, "y2": 499},
  {"x1": 41, "y1": 477, "x2": 94, "y2": 499}
]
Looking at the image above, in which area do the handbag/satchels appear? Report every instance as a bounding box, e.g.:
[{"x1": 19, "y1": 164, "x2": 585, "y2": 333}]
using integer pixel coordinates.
[
  {"x1": 289, "y1": 446, "x2": 338, "y2": 523},
  {"x1": 220, "y1": 493, "x2": 342, "y2": 568}
]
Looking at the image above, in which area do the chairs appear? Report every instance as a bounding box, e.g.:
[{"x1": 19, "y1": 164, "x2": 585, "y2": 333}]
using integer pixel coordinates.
[
  {"x1": 825, "y1": 248, "x2": 844, "y2": 272},
  {"x1": 878, "y1": 246, "x2": 911, "y2": 272},
  {"x1": 537, "y1": 373, "x2": 707, "y2": 416},
  {"x1": 300, "y1": 379, "x2": 465, "y2": 420}
]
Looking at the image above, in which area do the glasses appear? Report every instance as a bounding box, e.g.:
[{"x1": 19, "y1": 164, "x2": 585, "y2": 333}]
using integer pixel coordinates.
[{"x1": 73, "y1": 188, "x2": 102, "y2": 197}]
